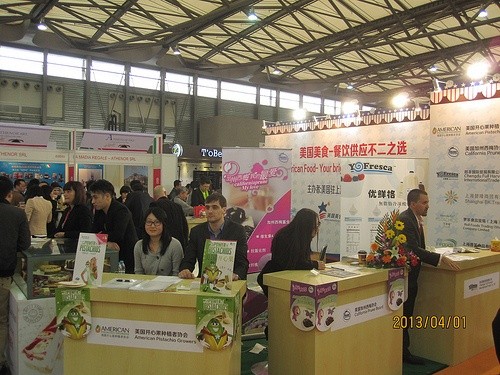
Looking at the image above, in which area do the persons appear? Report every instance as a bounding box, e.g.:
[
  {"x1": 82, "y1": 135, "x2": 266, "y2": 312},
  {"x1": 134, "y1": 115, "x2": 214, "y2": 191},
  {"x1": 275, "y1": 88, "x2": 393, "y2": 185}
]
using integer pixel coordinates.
[
  {"x1": 85, "y1": 180, "x2": 97, "y2": 210},
  {"x1": 25, "y1": 185, "x2": 53, "y2": 239},
  {"x1": 174, "y1": 185, "x2": 194, "y2": 215},
  {"x1": 0, "y1": 175, "x2": 31, "y2": 375},
  {"x1": 12, "y1": 179, "x2": 26, "y2": 206},
  {"x1": 168, "y1": 179, "x2": 199, "y2": 206},
  {"x1": 133, "y1": 207, "x2": 184, "y2": 275},
  {"x1": 123, "y1": 180, "x2": 156, "y2": 241},
  {"x1": 179, "y1": 192, "x2": 250, "y2": 301},
  {"x1": 396, "y1": 188, "x2": 461, "y2": 365},
  {"x1": 256, "y1": 208, "x2": 326, "y2": 341},
  {"x1": 54, "y1": 181, "x2": 93, "y2": 238},
  {"x1": 117, "y1": 186, "x2": 131, "y2": 204},
  {"x1": 153, "y1": 185, "x2": 189, "y2": 250},
  {"x1": 89, "y1": 179, "x2": 139, "y2": 274},
  {"x1": 192, "y1": 176, "x2": 212, "y2": 207},
  {"x1": 23, "y1": 179, "x2": 69, "y2": 240}
]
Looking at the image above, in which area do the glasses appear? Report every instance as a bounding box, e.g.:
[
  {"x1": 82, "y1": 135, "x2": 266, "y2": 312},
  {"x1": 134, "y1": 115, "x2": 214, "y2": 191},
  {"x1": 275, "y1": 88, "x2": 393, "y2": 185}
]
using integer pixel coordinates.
[{"x1": 145, "y1": 220, "x2": 161, "y2": 226}]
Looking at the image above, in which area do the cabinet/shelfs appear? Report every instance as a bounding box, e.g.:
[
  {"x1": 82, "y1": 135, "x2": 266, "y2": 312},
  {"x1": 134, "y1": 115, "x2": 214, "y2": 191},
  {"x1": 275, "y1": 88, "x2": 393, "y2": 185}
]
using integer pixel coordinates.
[
  {"x1": 12, "y1": 236, "x2": 119, "y2": 299},
  {"x1": 4, "y1": 281, "x2": 65, "y2": 375}
]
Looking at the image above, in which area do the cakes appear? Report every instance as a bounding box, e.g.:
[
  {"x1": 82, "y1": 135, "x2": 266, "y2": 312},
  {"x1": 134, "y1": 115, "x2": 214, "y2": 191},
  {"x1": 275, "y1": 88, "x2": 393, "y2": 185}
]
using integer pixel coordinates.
[{"x1": 39, "y1": 259, "x2": 76, "y2": 286}]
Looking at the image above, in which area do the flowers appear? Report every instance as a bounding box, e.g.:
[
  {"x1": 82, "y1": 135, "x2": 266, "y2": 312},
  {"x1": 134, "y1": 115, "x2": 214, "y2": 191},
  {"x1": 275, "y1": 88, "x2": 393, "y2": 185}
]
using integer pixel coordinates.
[{"x1": 365, "y1": 220, "x2": 420, "y2": 267}]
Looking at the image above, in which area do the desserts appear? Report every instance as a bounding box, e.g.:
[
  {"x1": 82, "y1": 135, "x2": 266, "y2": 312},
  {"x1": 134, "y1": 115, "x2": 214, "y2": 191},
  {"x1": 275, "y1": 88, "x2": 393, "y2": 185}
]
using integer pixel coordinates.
[{"x1": 22, "y1": 316, "x2": 63, "y2": 374}]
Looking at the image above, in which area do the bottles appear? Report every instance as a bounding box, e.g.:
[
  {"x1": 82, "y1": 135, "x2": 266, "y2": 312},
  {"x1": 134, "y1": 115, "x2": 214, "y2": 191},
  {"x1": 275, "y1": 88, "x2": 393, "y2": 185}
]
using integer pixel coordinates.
[{"x1": 119, "y1": 260, "x2": 126, "y2": 278}]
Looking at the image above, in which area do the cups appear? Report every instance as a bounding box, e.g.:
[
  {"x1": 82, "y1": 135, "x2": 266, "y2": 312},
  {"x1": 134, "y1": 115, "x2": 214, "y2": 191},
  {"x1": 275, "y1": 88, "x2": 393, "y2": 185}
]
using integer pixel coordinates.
[
  {"x1": 491, "y1": 240, "x2": 500, "y2": 252},
  {"x1": 358, "y1": 250, "x2": 367, "y2": 267}
]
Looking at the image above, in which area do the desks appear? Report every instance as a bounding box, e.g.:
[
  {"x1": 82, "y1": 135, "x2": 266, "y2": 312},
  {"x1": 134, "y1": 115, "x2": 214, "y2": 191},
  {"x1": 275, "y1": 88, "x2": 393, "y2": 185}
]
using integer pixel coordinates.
[
  {"x1": 262, "y1": 262, "x2": 407, "y2": 375},
  {"x1": 63, "y1": 269, "x2": 247, "y2": 375},
  {"x1": 408, "y1": 246, "x2": 500, "y2": 366}
]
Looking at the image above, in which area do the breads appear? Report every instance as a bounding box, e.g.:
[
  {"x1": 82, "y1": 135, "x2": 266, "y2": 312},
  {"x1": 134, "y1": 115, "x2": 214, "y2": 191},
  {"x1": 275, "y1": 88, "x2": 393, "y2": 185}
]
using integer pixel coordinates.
[{"x1": 228, "y1": 183, "x2": 275, "y2": 211}]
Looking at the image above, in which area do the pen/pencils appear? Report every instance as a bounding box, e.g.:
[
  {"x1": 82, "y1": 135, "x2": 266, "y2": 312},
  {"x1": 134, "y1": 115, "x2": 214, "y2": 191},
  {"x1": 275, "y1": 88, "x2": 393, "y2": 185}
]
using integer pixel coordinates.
[
  {"x1": 331, "y1": 266, "x2": 344, "y2": 270},
  {"x1": 116, "y1": 279, "x2": 131, "y2": 282}
]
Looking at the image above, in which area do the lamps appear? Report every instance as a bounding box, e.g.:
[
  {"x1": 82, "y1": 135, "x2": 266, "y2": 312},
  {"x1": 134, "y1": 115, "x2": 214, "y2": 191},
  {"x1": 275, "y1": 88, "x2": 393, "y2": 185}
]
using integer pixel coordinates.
[
  {"x1": 248, "y1": 6, "x2": 257, "y2": 20},
  {"x1": 432, "y1": 78, "x2": 441, "y2": 93},
  {"x1": 174, "y1": 44, "x2": 181, "y2": 55},
  {"x1": 346, "y1": 81, "x2": 353, "y2": 89},
  {"x1": 36, "y1": 17, "x2": 47, "y2": 31},
  {"x1": 478, "y1": 3, "x2": 488, "y2": 18},
  {"x1": 273, "y1": 64, "x2": 280, "y2": 74}
]
